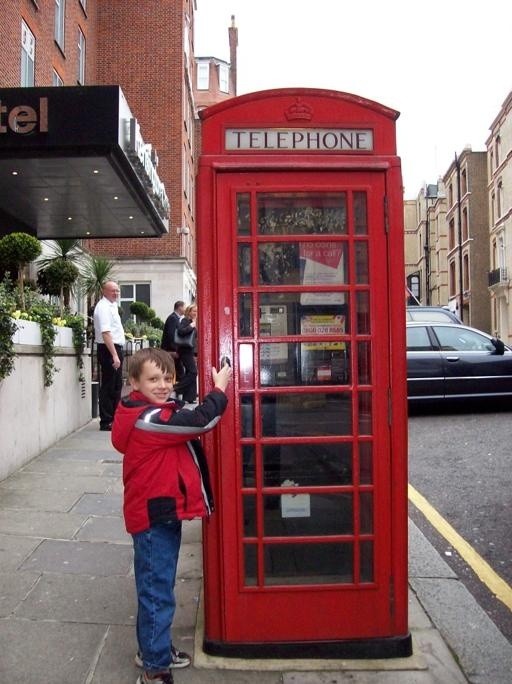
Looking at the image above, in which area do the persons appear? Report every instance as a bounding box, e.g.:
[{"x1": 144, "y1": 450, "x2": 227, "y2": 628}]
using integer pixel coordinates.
[
  {"x1": 92, "y1": 278, "x2": 127, "y2": 431},
  {"x1": 171, "y1": 304, "x2": 199, "y2": 405},
  {"x1": 160, "y1": 301, "x2": 186, "y2": 386},
  {"x1": 110, "y1": 344, "x2": 234, "y2": 684}
]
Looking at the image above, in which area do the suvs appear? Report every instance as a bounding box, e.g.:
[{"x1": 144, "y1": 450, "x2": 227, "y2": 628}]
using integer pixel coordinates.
[{"x1": 406, "y1": 305, "x2": 462, "y2": 325}]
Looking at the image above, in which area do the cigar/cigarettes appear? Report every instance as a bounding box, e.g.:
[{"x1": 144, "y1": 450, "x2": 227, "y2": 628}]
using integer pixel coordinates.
[{"x1": 115, "y1": 368, "x2": 117, "y2": 371}]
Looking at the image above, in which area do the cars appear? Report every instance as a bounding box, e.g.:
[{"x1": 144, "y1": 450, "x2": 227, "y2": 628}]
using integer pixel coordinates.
[{"x1": 406, "y1": 322, "x2": 512, "y2": 417}]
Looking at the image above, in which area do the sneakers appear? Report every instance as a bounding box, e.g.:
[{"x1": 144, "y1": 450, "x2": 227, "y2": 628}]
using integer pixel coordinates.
[
  {"x1": 136, "y1": 668, "x2": 174, "y2": 684},
  {"x1": 135, "y1": 640, "x2": 191, "y2": 669}
]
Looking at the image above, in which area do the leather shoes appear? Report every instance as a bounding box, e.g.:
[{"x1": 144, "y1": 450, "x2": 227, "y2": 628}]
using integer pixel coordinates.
[{"x1": 100, "y1": 423, "x2": 112, "y2": 431}]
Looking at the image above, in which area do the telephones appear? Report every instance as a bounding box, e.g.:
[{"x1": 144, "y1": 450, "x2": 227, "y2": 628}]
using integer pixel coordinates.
[{"x1": 242, "y1": 301, "x2": 297, "y2": 386}]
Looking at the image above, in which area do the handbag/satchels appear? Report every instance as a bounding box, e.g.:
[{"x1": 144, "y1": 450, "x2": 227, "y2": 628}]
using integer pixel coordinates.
[{"x1": 175, "y1": 329, "x2": 195, "y2": 348}]
